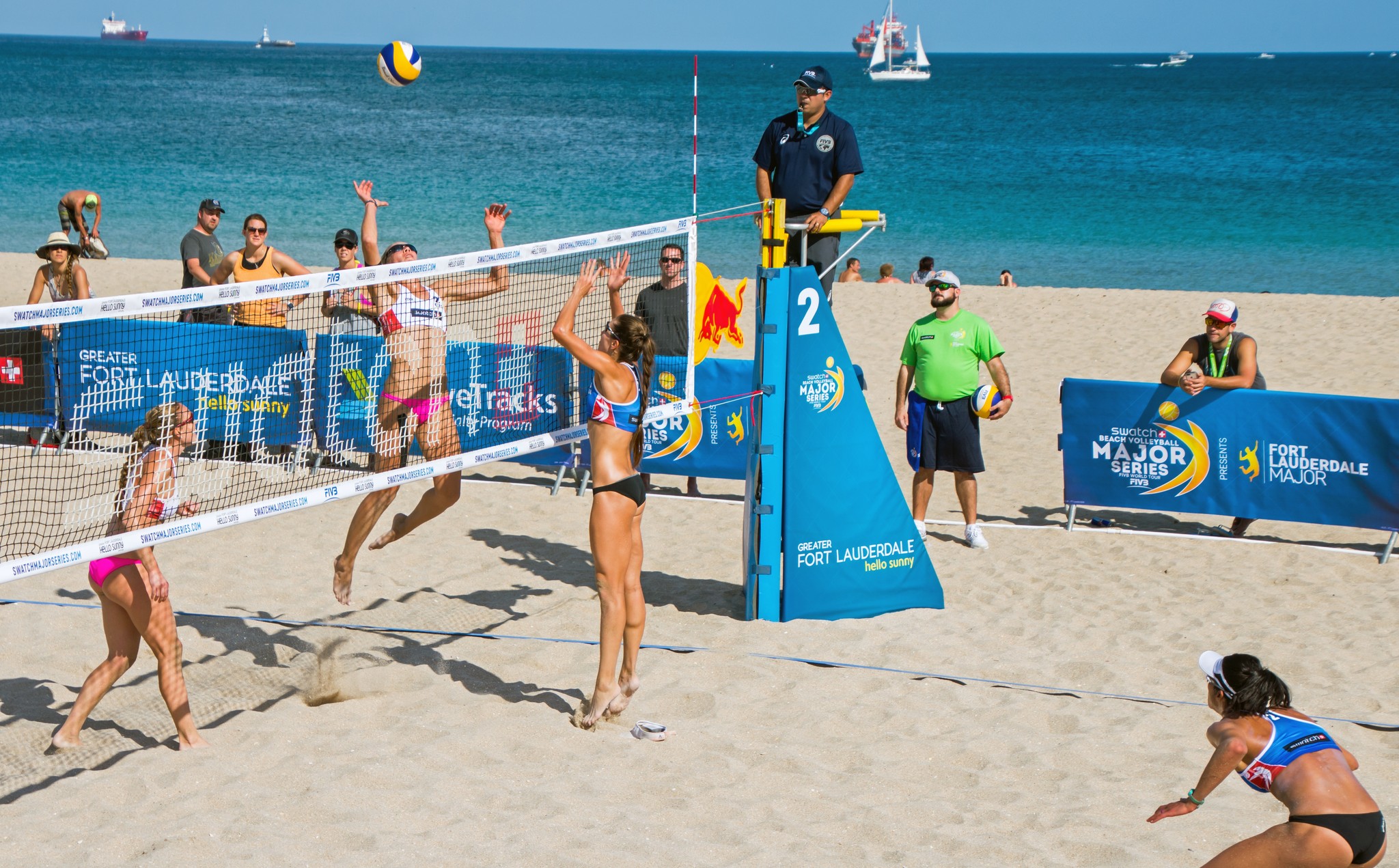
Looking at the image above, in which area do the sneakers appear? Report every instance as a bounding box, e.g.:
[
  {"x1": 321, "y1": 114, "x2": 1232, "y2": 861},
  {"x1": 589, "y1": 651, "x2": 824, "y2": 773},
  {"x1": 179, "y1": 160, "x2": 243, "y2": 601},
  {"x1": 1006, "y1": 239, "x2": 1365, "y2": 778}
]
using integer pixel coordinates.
[
  {"x1": 964, "y1": 525, "x2": 989, "y2": 549},
  {"x1": 914, "y1": 521, "x2": 927, "y2": 542}
]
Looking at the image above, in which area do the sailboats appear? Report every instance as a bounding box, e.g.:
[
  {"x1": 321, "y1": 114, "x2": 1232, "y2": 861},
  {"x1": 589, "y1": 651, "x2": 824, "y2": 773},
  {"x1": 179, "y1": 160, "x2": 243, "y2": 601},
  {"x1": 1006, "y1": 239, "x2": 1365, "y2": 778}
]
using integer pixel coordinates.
[{"x1": 862, "y1": 0, "x2": 930, "y2": 80}]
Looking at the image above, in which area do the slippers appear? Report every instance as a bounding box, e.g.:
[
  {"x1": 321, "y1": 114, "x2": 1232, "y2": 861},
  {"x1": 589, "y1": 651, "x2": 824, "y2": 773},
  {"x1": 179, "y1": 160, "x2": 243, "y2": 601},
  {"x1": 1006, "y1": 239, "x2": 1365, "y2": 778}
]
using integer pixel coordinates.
[
  {"x1": 279, "y1": 457, "x2": 293, "y2": 465},
  {"x1": 1186, "y1": 524, "x2": 1246, "y2": 537}
]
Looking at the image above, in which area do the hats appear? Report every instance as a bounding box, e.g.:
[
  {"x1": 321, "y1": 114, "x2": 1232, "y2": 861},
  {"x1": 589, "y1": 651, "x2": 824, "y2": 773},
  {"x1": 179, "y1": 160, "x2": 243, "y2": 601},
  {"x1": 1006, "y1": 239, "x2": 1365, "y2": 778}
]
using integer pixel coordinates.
[
  {"x1": 35, "y1": 232, "x2": 81, "y2": 261},
  {"x1": 1198, "y1": 651, "x2": 1237, "y2": 699},
  {"x1": 85, "y1": 194, "x2": 97, "y2": 212},
  {"x1": 200, "y1": 198, "x2": 225, "y2": 214},
  {"x1": 925, "y1": 270, "x2": 960, "y2": 289},
  {"x1": 332, "y1": 228, "x2": 358, "y2": 246},
  {"x1": 793, "y1": 66, "x2": 833, "y2": 91},
  {"x1": 1202, "y1": 299, "x2": 1238, "y2": 323}
]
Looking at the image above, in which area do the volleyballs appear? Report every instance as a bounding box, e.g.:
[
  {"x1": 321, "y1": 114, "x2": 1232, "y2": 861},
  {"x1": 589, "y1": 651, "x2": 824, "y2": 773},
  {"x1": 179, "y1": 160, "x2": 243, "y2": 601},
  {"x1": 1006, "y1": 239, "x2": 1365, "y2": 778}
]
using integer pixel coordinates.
[
  {"x1": 970, "y1": 385, "x2": 1002, "y2": 419},
  {"x1": 377, "y1": 41, "x2": 422, "y2": 86}
]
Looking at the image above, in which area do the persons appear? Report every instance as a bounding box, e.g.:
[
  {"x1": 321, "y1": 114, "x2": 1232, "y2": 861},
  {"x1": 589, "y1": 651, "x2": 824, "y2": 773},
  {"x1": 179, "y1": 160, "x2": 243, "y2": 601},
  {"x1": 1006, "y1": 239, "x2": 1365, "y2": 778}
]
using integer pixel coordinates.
[
  {"x1": 20, "y1": 181, "x2": 1017, "y2": 749},
  {"x1": 1160, "y1": 298, "x2": 1267, "y2": 534},
  {"x1": 753, "y1": 65, "x2": 865, "y2": 313},
  {"x1": 1146, "y1": 649, "x2": 1390, "y2": 868}
]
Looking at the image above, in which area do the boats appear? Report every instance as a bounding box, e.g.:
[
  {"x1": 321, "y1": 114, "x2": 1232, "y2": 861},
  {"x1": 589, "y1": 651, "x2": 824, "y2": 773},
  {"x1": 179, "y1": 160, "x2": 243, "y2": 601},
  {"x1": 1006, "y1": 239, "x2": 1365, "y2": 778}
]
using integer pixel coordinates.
[
  {"x1": 101, "y1": 11, "x2": 149, "y2": 41},
  {"x1": 852, "y1": 11, "x2": 909, "y2": 57},
  {"x1": 255, "y1": 23, "x2": 296, "y2": 47},
  {"x1": 1161, "y1": 50, "x2": 1276, "y2": 65}
]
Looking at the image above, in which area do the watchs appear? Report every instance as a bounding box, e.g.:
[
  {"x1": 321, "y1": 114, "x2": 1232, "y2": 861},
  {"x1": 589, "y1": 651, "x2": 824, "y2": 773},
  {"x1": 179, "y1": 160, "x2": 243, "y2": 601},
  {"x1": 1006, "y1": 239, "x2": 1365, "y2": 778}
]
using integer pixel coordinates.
[
  {"x1": 84, "y1": 237, "x2": 88, "y2": 240},
  {"x1": 285, "y1": 300, "x2": 294, "y2": 311},
  {"x1": 1002, "y1": 394, "x2": 1015, "y2": 402},
  {"x1": 819, "y1": 207, "x2": 831, "y2": 221},
  {"x1": 1187, "y1": 789, "x2": 1204, "y2": 807}
]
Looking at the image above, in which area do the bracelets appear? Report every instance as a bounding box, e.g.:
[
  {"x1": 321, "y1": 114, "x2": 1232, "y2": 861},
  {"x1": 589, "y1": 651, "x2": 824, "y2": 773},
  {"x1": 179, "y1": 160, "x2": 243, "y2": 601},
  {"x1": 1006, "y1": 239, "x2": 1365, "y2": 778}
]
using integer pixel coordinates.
[
  {"x1": 1000, "y1": 284, "x2": 1005, "y2": 286},
  {"x1": 227, "y1": 305, "x2": 232, "y2": 313},
  {"x1": 1178, "y1": 376, "x2": 1182, "y2": 388},
  {"x1": 365, "y1": 199, "x2": 379, "y2": 210}
]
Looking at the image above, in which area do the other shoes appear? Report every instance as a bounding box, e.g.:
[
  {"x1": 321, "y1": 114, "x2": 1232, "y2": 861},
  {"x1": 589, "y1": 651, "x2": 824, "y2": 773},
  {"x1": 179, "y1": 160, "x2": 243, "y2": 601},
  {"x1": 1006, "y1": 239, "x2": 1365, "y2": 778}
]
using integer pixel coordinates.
[{"x1": 307, "y1": 456, "x2": 349, "y2": 469}]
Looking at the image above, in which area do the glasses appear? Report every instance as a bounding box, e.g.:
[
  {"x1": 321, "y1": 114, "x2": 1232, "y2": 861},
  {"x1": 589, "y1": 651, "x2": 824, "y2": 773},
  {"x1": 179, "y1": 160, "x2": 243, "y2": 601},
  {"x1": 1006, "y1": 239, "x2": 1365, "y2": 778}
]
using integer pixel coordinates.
[
  {"x1": 335, "y1": 240, "x2": 357, "y2": 249},
  {"x1": 795, "y1": 85, "x2": 827, "y2": 96},
  {"x1": 1205, "y1": 317, "x2": 1233, "y2": 329},
  {"x1": 49, "y1": 245, "x2": 70, "y2": 250},
  {"x1": 929, "y1": 283, "x2": 958, "y2": 292},
  {"x1": 385, "y1": 244, "x2": 417, "y2": 264},
  {"x1": 164, "y1": 412, "x2": 194, "y2": 433},
  {"x1": 660, "y1": 257, "x2": 684, "y2": 263},
  {"x1": 604, "y1": 321, "x2": 622, "y2": 343},
  {"x1": 1206, "y1": 674, "x2": 1234, "y2": 696},
  {"x1": 246, "y1": 226, "x2": 267, "y2": 236}
]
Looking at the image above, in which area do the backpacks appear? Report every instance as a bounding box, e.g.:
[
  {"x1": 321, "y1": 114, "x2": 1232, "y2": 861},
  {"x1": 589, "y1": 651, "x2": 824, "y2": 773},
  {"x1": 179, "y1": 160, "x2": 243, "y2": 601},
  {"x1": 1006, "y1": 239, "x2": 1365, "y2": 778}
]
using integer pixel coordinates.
[{"x1": 77, "y1": 231, "x2": 111, "y2": 260}]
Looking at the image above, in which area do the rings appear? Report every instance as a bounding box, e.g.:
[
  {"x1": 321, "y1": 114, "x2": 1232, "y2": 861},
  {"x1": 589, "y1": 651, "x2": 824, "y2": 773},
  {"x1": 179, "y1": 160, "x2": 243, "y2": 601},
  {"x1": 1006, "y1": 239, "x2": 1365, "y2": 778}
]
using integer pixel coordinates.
[
  {"x1": 816, "y1": 223, "x2": 819, "y2": 225},
  {"x1": 276, "y1": 309, "x2": 278, "y2": 312}
]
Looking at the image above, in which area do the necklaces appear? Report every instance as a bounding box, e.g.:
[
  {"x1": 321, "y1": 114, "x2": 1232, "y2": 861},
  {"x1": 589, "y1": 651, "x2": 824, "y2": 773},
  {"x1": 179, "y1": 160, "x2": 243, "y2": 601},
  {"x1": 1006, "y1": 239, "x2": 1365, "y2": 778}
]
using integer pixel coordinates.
[{"x1": 247, "y1": 244, "x2": 264, "y2": 269}]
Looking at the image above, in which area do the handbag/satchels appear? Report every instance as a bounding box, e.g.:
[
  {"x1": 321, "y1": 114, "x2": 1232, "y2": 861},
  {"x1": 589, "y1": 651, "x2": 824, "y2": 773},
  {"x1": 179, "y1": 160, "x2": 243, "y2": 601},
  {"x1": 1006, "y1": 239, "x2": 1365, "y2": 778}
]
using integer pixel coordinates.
[{"x1": 202, "y1": 439, "x2": 252, "y2": 463}]
